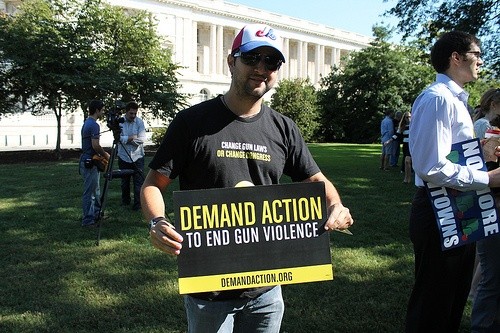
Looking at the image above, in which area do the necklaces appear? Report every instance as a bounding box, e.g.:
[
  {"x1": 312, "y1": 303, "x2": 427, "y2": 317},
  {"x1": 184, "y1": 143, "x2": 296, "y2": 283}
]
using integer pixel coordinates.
[{"x1": 223, "y1": 95, "x2": 236, "y2": 115}]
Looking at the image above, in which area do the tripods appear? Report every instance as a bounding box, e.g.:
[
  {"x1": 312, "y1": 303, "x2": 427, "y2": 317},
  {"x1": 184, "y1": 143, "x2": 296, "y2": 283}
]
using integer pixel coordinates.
[{"x1": 94, "y1": 137, "x2": 175, "y2": 246}]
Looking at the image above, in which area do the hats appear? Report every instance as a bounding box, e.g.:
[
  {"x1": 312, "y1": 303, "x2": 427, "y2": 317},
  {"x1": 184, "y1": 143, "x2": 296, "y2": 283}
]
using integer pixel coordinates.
[{"x1": 232, "y1": 24, "x2": 285, "y2": 63}]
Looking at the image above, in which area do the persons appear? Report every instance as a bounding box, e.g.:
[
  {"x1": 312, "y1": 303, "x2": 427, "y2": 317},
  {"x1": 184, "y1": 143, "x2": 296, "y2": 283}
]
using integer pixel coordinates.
[
  {"x1": 392, "y1": 111, "x2": 403, "y2": 168},
  {"x1": 468, "y1": 88, "x2": 500, "y2": 333},
  {"x1": 380, "y1": 109, "x2": 395, "y2": 172},
  {"x1": 139, "y1": 23, "x2": 354, "y2": 333},
  {"x1": 79, "y1": 100, "x2": 110, "y2": 227},
  {"x1": 406, "y1": 32, "x2": 500, "y2": 333},
  {"x1": 114, "y1": 102, "x2": 146, "y2": 210},
  {"x1": 384, "y1": 111, "x2": 413, "y2": 183}
]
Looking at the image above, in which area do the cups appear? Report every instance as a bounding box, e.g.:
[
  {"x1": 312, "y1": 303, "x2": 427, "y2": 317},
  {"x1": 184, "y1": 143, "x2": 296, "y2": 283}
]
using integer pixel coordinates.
[{"x1": 483, "y1": 128, "x2": 500, "y2": 163}]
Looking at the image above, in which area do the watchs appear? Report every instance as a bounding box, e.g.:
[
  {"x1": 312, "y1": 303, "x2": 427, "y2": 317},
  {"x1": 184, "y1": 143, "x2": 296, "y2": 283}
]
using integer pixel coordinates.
[{"x1": 147, "y1": 217, "x2": 167, "y2": 234}]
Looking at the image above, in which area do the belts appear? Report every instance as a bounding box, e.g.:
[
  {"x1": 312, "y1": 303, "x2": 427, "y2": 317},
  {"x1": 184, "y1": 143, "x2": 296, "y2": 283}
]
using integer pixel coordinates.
[{"x1": 418, "y1": 186, "x2": 428, "y2": 193}]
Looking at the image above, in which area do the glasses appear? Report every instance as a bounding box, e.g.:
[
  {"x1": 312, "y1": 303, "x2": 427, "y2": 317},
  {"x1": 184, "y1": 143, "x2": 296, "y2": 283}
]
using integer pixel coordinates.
[
  {"x1": 234, "y1": 51, "x2": 281, "y2": 71},
  {"x1": 466, "y1": 51, "x2": 484, "y2": 60}
]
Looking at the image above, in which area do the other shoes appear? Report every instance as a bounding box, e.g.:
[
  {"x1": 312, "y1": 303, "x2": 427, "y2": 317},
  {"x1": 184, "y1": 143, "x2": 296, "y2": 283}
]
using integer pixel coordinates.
[
  {"x1": 80, "y1": 216, "x2": 101, "y2": 225},
  {"x1": 132, "y1": 206, "x2": 141, "y2": 213},
  {"x1": 119, "y1": 200, "x2": 130, "y2": 205},
  {"x1": 384, "y1": 167, "x2": 390, "y2": 172},
  {"x1": 380, "y1": 166, "x2": 384, "y2": 171}
]
